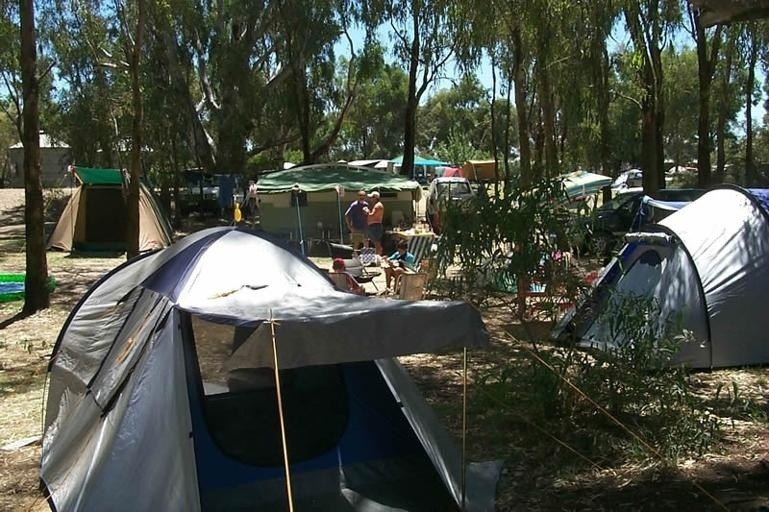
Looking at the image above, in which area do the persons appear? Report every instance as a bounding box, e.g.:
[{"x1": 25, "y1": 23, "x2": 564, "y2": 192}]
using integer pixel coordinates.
[
  {"x1": 332, "y1": 257, "x2": 366, "y2": 295},
  {"x1": 380, "y1": 240, "x2": 415, "y2": 298},
  {"x1": 343, "y1": 190, "x2": 371, "y2": 249},
  {"x1": 246, "y1": 178, "x2": 257, "y2": 214},
  {"x1": 362, "y1": 191, "x2": 385, "y2": 255}
]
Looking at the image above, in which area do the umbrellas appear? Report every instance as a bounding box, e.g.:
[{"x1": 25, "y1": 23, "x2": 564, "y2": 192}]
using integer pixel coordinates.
[{"x1": 530, "y1": 169, "x2": 613, "y2": 254}]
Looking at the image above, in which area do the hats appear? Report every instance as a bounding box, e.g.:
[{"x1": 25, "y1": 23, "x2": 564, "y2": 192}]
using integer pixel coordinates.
[
  {"x1": 367, "y1": 191, "x2": 380, "y2": 199},
  {"x1": 334, "y1": 258, "x2": 345, "y2": 266}
]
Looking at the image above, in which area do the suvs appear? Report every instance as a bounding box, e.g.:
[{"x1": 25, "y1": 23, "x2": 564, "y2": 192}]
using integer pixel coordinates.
[
  {"x1": 570, "y1": 187, "x2": 712, "y2": 263},
  {"x1": 172, "y1": 166, "x2": 220, "y2": 216}
]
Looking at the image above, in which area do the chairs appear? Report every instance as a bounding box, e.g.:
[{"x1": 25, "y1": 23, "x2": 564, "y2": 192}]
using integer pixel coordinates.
[{"x1": 281, "y1": 221, "x2": 440, "y2": 300}]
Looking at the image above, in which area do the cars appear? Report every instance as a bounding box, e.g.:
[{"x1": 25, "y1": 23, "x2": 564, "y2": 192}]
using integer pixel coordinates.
[{"x1": 422, "y1": 175, "x2": 478, "y2": 233}]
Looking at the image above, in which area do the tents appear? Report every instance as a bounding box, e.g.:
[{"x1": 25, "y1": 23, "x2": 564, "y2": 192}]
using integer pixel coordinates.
[
  {"x1": 7, "y1": 128, "x2": 71, "y2": 189},
  {"x1": 463, "y1": 158, "x2": 497, "y2": 182},
  {"x1": 255, "y1": 161, "x2": 424, "y2": 235},
  {"x1": 745, "y1": 189, "x2": 768, "y2": 255},
  {"x1": 47, "y1": 165, "x2": 174, "y2": 255},
  {"x1": 33, "y1": 221, "x2": 492, "y2": 511},
  {"x1": 555, "y1": 184, "x2": 768, "y2": 373}
]
